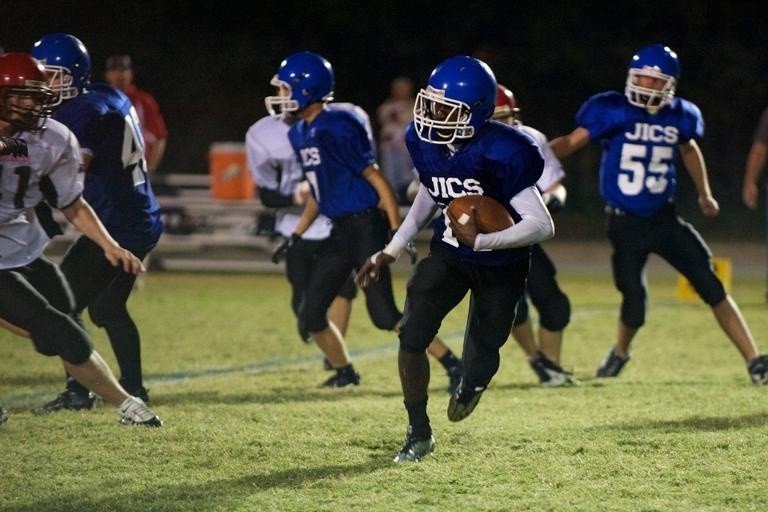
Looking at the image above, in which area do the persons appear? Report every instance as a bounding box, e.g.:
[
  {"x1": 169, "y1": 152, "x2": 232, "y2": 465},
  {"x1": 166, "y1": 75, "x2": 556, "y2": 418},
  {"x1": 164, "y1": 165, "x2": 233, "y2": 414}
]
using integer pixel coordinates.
[
  {"x1": 244, "y1": 100, "x2": 357, "y2": 370},
  {"x1": 742, "y1": 102, "x2": 767, "y2": 213},
  {"x1": 373, "y1": 73, "x2": 420, "y2": 206},
  {"x1": 98, "y1": 53, "x2": 165, "y2": 293},
  {"x1": 29, "y1": 33, "x2": 165, "y2": 411},
  {"x1": 269, "y1": 51, "x2": 465, "y2": 394},
  {"x1": 0, "y1": 50, "x2": 166, "y2": 428},
  {"x1": 487, "y1": 83, "x2": 579, "y2": 388},
  {"x1": 549, "y1": 42, "x2": 768, "y2": 390},
  {"x1": 353, "y1": 53, "x2": 554, "y2": 464}
]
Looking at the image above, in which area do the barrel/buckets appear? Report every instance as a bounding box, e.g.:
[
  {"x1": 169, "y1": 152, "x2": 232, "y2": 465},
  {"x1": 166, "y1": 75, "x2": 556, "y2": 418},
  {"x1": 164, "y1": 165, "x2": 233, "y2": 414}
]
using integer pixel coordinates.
[{"x1": 209, "y1": 141, "x2": 255, "y2": 200}]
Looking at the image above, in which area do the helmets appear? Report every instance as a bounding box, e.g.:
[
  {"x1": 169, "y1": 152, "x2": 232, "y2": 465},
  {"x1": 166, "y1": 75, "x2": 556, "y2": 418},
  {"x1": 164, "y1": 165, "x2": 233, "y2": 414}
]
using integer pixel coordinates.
[
  {"x1": 265, "y1": 51, "x2": 335, "y2": 125},
  {"x1": 414, "y1": 55, "x2": 519, "y2": 144},
  {"x1": 0, "y1": 33, "x2": 92, "y2": 131},
  {"x1": 624, "y1": 46, "x2": 679, "y2": 110}
]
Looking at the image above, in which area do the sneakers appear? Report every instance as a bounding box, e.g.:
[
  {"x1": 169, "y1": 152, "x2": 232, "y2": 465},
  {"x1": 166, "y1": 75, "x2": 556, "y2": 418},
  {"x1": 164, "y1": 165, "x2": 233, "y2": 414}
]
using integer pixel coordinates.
[
  {"x1": 315, "y1": 356, "x2": 360, "y2": 387},
  {"x1": 115, "y1": 380, "x2": 164, "y2": 426},
  {"x1": 598, "y1": 345, "x2": 630, "y2": 377},
  {"x1": 747, "y1": 354, "x2": 768, "y2": 387},
  {"x1": 528, "y1": 350, "x2": 583, "y2": 386},
  {"x1": 42, "y1": 386, "x2": 94, "y2": 410},
  {"x1": 393, "y1": 424, "x2": 436, "y2": 464},
  {"x1": 446, "y1": 360, "x2": 486, "y2": 421}
]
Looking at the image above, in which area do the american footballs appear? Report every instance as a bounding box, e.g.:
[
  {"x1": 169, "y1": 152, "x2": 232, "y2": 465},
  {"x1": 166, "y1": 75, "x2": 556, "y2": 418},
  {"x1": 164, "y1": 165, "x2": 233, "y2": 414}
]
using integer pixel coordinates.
[{"x1": 444, "y1": 196, "x2": 514, "y2": 234}]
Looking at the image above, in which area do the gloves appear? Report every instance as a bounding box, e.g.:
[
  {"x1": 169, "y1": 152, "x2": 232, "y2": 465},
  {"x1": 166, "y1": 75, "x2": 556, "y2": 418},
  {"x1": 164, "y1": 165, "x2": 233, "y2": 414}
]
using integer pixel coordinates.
[
  {"x1": 390, "y1": 229, "x2": 418, "y2": 265},
  {"x1": 270, "y1": 232, "x2": 301, "y2": 263}
]
